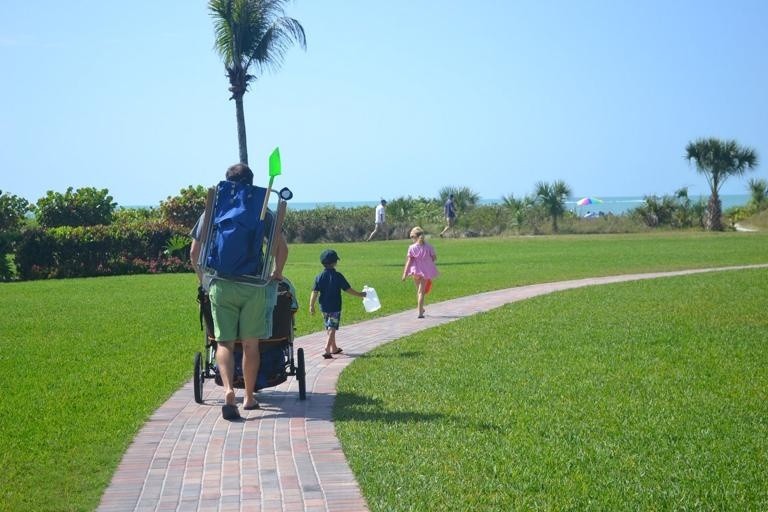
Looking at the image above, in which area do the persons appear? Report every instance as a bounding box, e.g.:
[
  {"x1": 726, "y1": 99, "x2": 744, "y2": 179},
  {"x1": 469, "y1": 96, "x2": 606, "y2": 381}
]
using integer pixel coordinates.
[
  {"x1": 439, "y1": 194, "x2": 459, "y2": 239},
  {"x1": 584, "y1": 211, "x2": 592, "y2": 217},
  {"x1": 186, "y1": 162, "x2": 289, "y2": 421},
  {"x1": 402, "y1": 226, "x2": 438, "y2": 318},
  {"x1": 309, "y1": 249, "x2": 367, "y2": 359},
  {"x1": 366, "y1": 199, "x2": 391, "y2": 243}
]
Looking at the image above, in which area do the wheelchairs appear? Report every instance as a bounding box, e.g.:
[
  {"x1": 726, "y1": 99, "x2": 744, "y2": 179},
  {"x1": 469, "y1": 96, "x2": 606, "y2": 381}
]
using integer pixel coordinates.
[{"x1": 192, "y1": 281, "x2": 307, "y2": 403}]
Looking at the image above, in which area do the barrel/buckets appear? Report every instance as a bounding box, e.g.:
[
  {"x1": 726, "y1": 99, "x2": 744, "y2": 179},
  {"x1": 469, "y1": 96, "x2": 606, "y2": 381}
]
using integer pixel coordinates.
[{"x1": 360, "y1": 285, "x2": 381, "y2": 312}]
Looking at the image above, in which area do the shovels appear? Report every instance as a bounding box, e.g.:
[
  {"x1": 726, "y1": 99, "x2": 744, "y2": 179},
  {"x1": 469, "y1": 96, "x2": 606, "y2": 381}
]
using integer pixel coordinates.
[{"x1": 260, "y1": 147, "x2": 282, "y2": 221}]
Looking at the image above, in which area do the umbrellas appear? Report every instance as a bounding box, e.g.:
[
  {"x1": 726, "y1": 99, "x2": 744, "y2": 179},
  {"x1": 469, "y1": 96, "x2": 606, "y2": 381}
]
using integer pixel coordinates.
[{"x1": 576, "y1": 197, "x2": 604, "y2": 207}]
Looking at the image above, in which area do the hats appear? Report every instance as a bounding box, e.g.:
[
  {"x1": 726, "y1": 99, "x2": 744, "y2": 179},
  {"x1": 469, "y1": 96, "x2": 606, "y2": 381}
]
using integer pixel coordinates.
[{"x1": 320, "y1": 250, "x2": 340, "y2": 264}]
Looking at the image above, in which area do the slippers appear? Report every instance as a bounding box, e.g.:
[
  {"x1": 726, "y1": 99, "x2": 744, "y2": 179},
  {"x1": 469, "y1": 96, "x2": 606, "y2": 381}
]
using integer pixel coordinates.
[
  {"x1": 222, "y1": 405, "x2": 240, "y2": 419},
  {"x1": 243, "y1": 401, "x2": 259, "y2": 410},
  {"x1": 322, "y1": 348, "x2": 342, "y2": 358}
]
